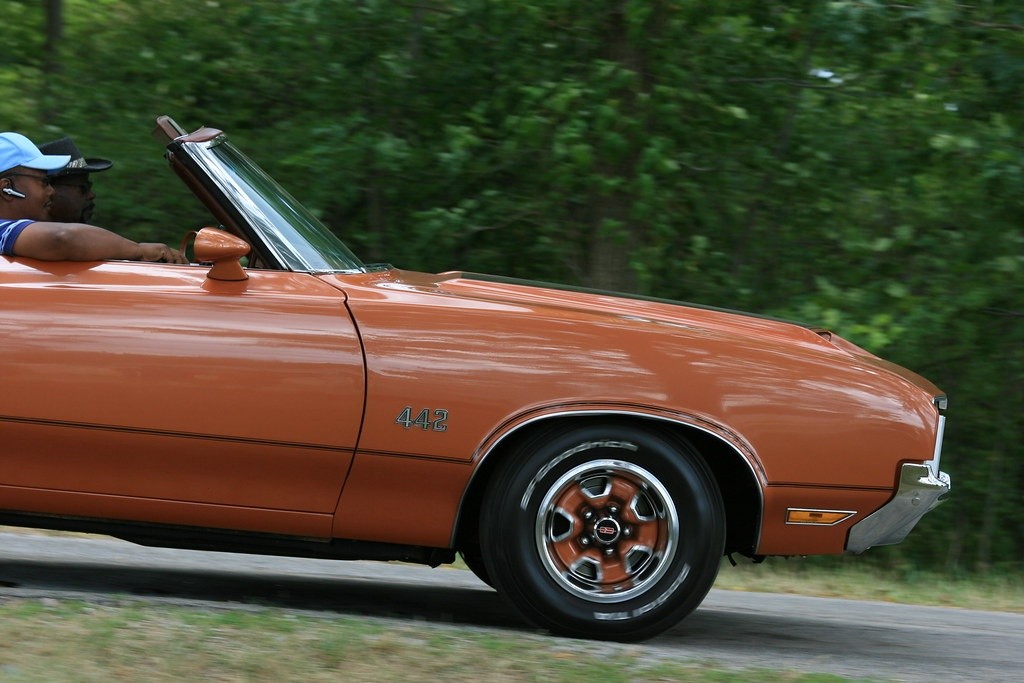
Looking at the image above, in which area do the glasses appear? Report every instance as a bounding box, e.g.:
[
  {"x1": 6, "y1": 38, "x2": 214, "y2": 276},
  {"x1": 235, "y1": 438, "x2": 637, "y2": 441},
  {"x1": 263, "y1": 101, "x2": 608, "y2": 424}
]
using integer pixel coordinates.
[
  {"x1": 1, "y1": 172, "x2": 52, "y2": 187},
  {"x1": 53, "y1": 181, "x2": 93, "y2": 196},
  {"x1": 9, "y1": 174, "x2": 49, "y2": 189}
]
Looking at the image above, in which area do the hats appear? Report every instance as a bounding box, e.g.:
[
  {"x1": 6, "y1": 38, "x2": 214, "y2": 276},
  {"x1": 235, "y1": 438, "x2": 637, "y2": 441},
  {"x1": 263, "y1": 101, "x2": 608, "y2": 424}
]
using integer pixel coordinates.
[
  {"x1": 37, "y1": 136, "x2": 113, "y2": 172},
  {"x1": 0, "y1": 131, "x2": 71, "y2": 173}
]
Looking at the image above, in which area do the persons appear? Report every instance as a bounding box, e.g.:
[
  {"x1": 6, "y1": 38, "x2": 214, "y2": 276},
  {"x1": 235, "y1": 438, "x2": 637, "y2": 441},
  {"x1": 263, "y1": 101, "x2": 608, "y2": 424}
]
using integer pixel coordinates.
[
  {"x1": 35, "y1": 138, "x2": 112, "y2": 222},
  {"x1": 0, "y1": 131, "x2": 189, "y2": 264}
]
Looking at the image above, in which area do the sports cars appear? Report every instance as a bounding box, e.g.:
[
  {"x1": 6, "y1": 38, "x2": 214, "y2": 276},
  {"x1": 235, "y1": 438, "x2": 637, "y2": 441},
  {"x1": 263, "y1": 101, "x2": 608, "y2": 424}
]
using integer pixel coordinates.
[{"x1": 0, "y1": 112, "x2": 956, "y2": 641}]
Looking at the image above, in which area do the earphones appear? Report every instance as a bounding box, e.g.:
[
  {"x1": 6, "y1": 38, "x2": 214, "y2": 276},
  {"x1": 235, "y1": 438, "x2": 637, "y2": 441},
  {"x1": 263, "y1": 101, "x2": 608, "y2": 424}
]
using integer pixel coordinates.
[{"x1": 3, "y1": 188, "x2": 26, "y2": 199}]
